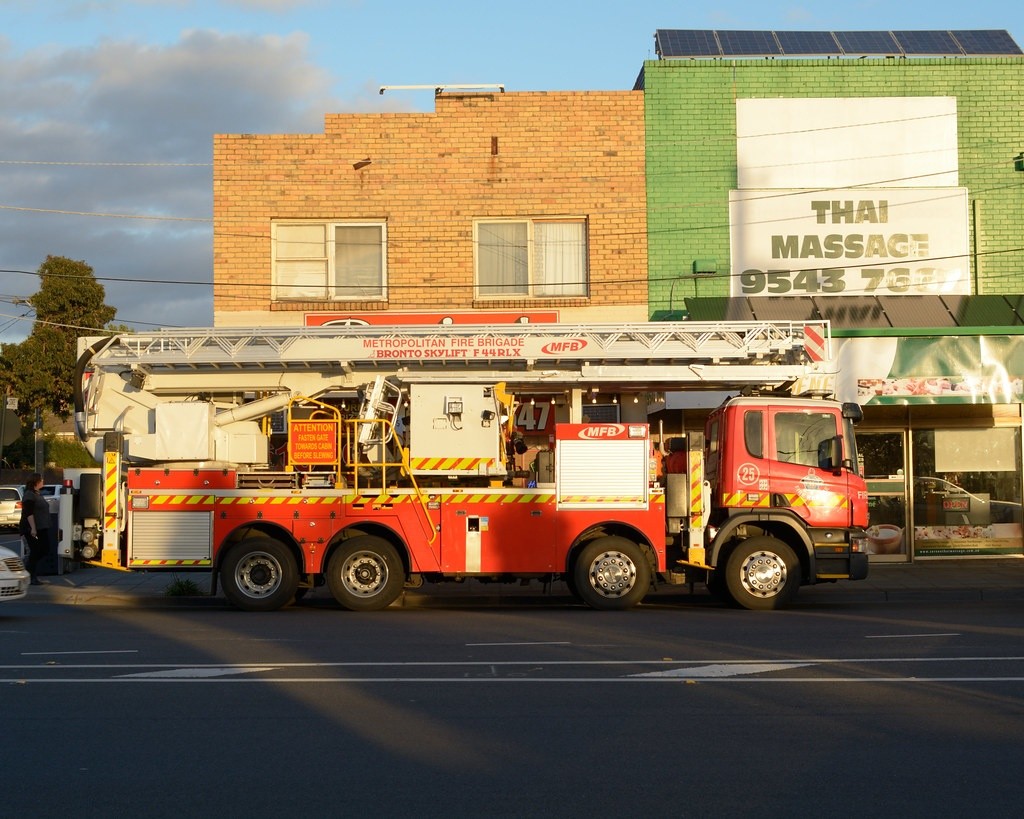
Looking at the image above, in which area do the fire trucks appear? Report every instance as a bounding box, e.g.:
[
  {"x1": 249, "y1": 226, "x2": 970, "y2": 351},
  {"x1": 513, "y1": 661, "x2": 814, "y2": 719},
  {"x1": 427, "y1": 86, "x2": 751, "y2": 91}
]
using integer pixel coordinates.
[{"x1": 57, "y1": 320, "x2": 870, "y2": 611}]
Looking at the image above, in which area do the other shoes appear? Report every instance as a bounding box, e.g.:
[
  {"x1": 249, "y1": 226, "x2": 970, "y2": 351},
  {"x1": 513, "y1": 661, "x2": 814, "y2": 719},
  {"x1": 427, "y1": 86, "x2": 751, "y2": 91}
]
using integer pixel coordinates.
[{"x1": 31, "y1": 579, "x2": 42, "y2": 586}]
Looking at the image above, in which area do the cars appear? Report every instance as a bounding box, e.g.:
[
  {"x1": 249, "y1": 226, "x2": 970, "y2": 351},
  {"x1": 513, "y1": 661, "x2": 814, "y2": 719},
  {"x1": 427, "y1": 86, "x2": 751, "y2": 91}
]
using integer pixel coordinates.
[
  {"x1": 1, "y1": 485, "x2": 26, "y2": 528},
  {"x1": 40, "y1": 485, "x2": 62, "y2": 514},
  {"x1": 0, "y1": 545, "x2": 30, "y2": 602},
  {"x1": 917, "y1": 476, "x2": 1023, "y2": 522}
]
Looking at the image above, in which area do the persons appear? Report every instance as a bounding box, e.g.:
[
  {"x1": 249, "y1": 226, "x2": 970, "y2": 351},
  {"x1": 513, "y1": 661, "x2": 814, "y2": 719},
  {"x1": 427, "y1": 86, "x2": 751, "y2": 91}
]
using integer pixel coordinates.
[{"x1": 18, "y1": 473, "x2": 49, "y2": 585}]
